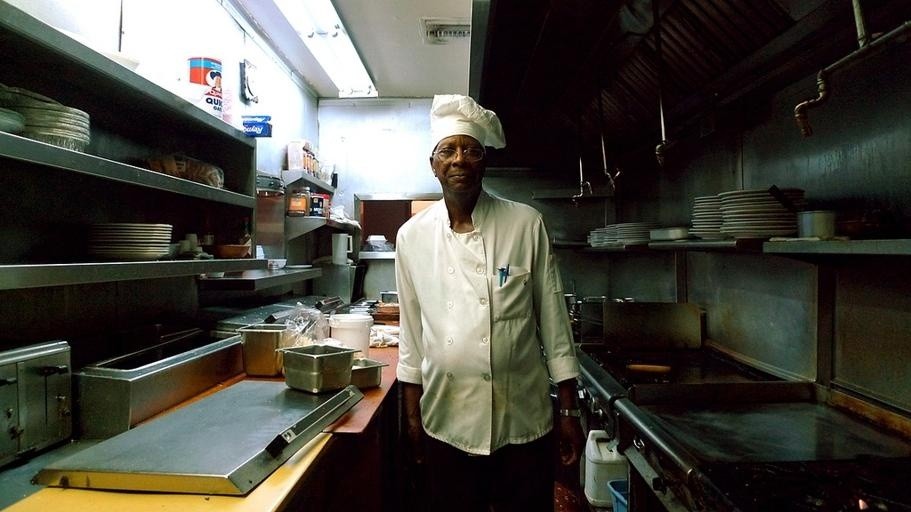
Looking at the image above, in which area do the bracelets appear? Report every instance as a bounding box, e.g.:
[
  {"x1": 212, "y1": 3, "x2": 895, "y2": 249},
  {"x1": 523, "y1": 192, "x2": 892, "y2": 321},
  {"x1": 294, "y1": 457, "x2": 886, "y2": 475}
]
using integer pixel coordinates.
[{"x1": 557, "y1": 405, "x2": 585, "y2": 420}]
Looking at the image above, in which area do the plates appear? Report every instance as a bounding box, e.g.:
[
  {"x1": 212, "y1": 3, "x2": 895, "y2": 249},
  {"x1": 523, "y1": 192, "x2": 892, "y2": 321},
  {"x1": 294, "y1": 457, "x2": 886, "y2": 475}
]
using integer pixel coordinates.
[
  {"x1": 0, "y1": 87, "x2": 90, "y2": 152},
  {"x1": 615, "y1": 223, "x2": 654, "y2": 245},
  {"x1": 0, "y1": 105, "x2": 25, "y2": 135},
  {"x1": 85, "y1": 222, "x2": 173, "y2": 261},
  {"x1": 589, "y1": 223, "x2": 617, "y2": 246},
  {"x1": 285, "y1": 265, "x2": 312, "y2": 269},
  {"x1": 687, "y1": 196, "x2": 727, "y2": 240},
  {"x1": 717, "y1": 188, "x2": 804, "y2": 239}
]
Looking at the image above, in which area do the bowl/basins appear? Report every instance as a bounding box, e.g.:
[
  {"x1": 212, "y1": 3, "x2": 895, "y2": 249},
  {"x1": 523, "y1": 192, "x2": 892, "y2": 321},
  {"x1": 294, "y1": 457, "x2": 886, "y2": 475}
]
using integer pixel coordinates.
[
  {"x1": 267, "y1": 259, "x2": 286, "y2": 269},
  {"x1": 214, "y1": 244, "x2": 248, "y2": 258}
]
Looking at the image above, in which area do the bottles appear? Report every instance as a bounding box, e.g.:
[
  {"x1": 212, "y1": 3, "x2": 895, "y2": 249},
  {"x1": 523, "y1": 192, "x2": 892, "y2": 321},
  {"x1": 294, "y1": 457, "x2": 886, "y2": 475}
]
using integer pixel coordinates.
[
  {"x1": 287, "y1": 183, "x2": 330, "y2": 217},
  {"x1": 239, "y1": 217, "x2": 252, "y2": 258}
]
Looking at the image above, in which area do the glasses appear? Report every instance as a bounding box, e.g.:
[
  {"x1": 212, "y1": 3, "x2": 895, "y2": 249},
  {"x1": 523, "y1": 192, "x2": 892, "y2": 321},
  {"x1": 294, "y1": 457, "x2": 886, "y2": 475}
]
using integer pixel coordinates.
[{"x1": 432, "y1": 148, "x2": 483, "y2": 162}]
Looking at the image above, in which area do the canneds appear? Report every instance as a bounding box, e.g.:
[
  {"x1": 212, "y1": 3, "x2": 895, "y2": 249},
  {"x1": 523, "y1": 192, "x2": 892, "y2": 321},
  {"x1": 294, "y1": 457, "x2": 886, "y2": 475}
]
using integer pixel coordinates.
[
  {"x1": 308, "y1": 196, "x2": 324, "y2": 216},
  {"x1": 287, "y1": 193, "x2": 305, "y2": 217}
]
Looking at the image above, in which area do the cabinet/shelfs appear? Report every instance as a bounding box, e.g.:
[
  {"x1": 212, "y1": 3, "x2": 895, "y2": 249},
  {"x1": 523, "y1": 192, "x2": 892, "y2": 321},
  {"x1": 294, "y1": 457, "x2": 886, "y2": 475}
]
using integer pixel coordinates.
[
  {"x1": 1, "y1": 3, "x2": 259, "y2": 291},
  {"x1": 284, "y1": 168, "x2": 362, "y2": 243}
]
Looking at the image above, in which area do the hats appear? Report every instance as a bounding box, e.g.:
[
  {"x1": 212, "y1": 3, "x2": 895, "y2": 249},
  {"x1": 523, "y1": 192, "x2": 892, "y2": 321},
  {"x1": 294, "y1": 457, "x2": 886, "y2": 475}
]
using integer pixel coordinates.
[{"x1": 430, "y1": 94, "x2": 507, "y2": 157}]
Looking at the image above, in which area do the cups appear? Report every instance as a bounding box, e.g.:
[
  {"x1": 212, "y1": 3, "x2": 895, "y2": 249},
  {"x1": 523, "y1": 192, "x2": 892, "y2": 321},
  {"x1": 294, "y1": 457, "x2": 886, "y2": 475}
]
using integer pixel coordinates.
[
  {"x1": 178, "y1": 232, "x2": 202, "y2": 259},
  {"x1": 331, "y1": 233, "x2": 352, "y2": 265}
]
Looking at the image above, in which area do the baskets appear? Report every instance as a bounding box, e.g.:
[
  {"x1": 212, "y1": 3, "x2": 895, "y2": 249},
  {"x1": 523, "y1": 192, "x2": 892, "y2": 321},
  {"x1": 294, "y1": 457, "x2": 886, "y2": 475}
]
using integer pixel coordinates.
[{"x1": 606, "y1": 479, "x2": 628, "y2": 512}]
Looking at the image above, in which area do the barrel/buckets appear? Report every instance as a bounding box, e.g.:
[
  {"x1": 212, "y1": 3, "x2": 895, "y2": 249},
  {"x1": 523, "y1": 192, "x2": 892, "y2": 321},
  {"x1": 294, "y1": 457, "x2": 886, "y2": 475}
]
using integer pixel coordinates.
[
  {"x1": 330, "y1": 313, "x2": 375, "y2": 358},
  {"x1": 583, "y1": 430, "x2": 630, "y2": 507}
]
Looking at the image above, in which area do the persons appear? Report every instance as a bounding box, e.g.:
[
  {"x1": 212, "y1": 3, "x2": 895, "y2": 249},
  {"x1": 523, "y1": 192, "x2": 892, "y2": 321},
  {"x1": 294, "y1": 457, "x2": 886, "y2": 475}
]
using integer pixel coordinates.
[
  {"x1": 209, "y1": 70, "x2": 223, "y2": 92},
  {"x1": 393, "y1": 92, "x2": 589, "y2": 508}
]
